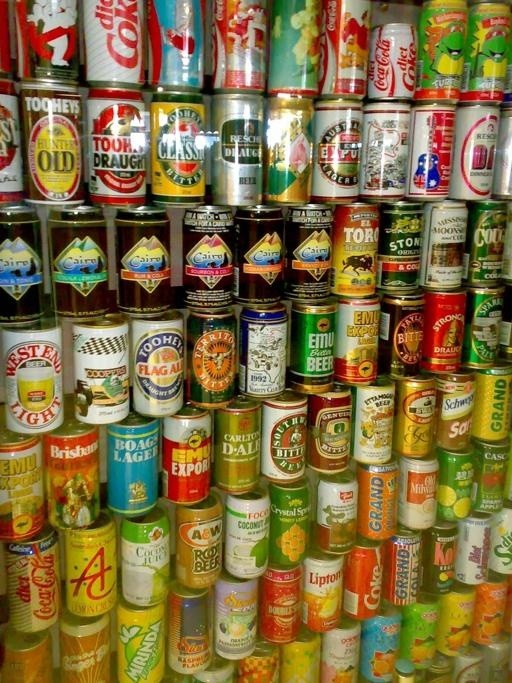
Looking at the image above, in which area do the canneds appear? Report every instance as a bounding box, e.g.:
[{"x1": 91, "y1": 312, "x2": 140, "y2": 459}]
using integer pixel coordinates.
[
  {"x1": 148, "y1": 89, "x2": 207, "y2": 209},
  {"x1": 320, "y1": 0, "x2": 373, "y2": 100},
  {"x1": 166, "y1": 586, "x2": 211, "y2": 677},
  {"x1": 270, "y1": 481, "x2": 311, "y2": 567},
  {"x1": 379, "y1": 293, "x2": 427, "y2": 381},
  {"x1": 395, "y1": 372, "x2": 437, "y2": 460},
  {"x1": 48, "y1": 417, "x2": 101, "y2": 533},
  {"x1": 352, "y1": 375, "x2": 395, "y2": 466},
  {"x1": 267, "y1": 0, "x2": 323, "y2": 99},
  {"x1": 261, "y1": 391, "x2": 310, "y2": 485},
  {"x1": 213, "y1": 397, "x2": 262, "y2": 495},
  {"x1": 0, "y1": 434, "x2": 44, "y2": 545},
  {"x1": 301, "y1": 553, "x2": 345, "y2": 633},
  {"x1": 397, "y1": 456, "x2": 440, "y2": 531},
  {"x1": 116, "y1": 505, "x2": 170, "y2": 608},
  {"x1": 485, "y1": 632, "x2": 511, "y2": 682},
  {"x1": 232, "y1": 205, "x2": 286, "y2": 309},
  {"x1": 114, "y1": 205, "x2": 172, "y2": 320},
  {"x1": 81, "y1": 0, "x2": 146, "y2": 90},
  {"x1": 419, "y1": 291, "x2": 467, "y2": 375},
  {"x1": 376, "y1": 202, "x2": 425, "y2": 298},
  {"x1": 329, "y1": 202, "x2": 379, "y2": 299},
  {"x1": 306, "y1": 384, "x2": 353, "y2": 475},
  {"x1": 491, "y1": 105, "x2": 512, "y2": 202},
  {"x1": 395, "y1": 659, "x2": 418, "y2": 683},
  {"x1": 233, "y1": 643, "x2": 279, "y2": 683},
  {"x1": 290, "y1": 298, "x2": 338, "y2": 396},
  {"x1": 316, "y1": 468, "x2": 360, "y2": 554},
  {"x1": 259, "y1": 567, "x2": 304, "y2": 643},
  {"x1": 462, "y1": 288, "x2": 508, "y2": 371},
  {"x1": 370, "y1": 23, "x2": 419, "y2": 100},
  {"x1": 0, "y1": 324, "x2": 66, "y2": 437},
  {"x1": 70, "y1": 316, "x2": 130, "y2": 427},
  {"x1": 0, "y1": 0, "x2": 12, "y2": 78},
  {"x1": 447, "y1": 105, "x2": 500, "y2": 203},
  {"x1": 452, "y1": 649, "x2": 484, "y2": 681},
  {"x1": 0, "y1": 80, "x2": 28, "y2": 205},
  {"x1": 407, "y1": 103, "x2": 457, "y2": 203},
  {"x1": 503, "y1": 62, "x2": 512, "y2": 104},
  {"x1": 115, "y1": 603, "x2": 166, "y2": 683},
  {"x1": 106, "y1": 411, "x2": 161, "y2": 518},
  {"x1": 86, "y1": 91, "x2": 147, "y2": 208},
  {"x1": 486, "y1": 504, "x2": 512, "y2": 574},
  {"x1": 189, "y1": 661, "x2": 233, "y2": 682},
  {"x1": 211, "y1": 0, "x2": 270, "y2": 94},
  {"x1": 313, "y1": 96, "x2": 363, "y2": 207},
  {"x1": 361, "y1": 103, "x2": 413, "y2": 205},
  {"x1": 147, "y1": 0, "x2": 206, "y2": 92},
  {"x1": 434, "y1": 449, "x2": 477, "y2": 522},
  {"x1": 356, "y1": 462, "x2": 400, "y2": 542},
  {"x1": 438, "y1": 588, "x2": 476, "y2": 656},
  {"x1": 501, "y1": 201, "x2": 512, "y2": 286},
  {"x1": 424, "y1": 523, "x2": 459, "y2": 592},
  {"x1": 499, "y1": 319, "x2": 512, "y2": 368},
  {"x1": 426, "y1": 657, "x2": 452, "y2": 683},
  {"x1": 342, "y1": 544, "x2": 386, "y2": 619},
  {"x1": 20, "y1": 82, "x2": 86, "y2": 207},
  {"x1": 464, "y1": 1, "x2": 510, "y2": 105},
  {"x1": 415, "y1": 0, "x2": 468, "y2": 103},
  {"x1": 418, "y1": 201, "x2": 470, "y2": 292},
  {"x1": 3, "y1": 530, "x2": 61, "y2": 634},
  {"x1": 59, "y1": 508, "x2": 118, "y2": 619},
  {"x1": 175, "y1": 495, "x2": 224, "y2": 590},
  {"x1": 181, "y1": 203, "x2": 234, "y2": 312},
  {"x1": 1, "y1": 204, "x2": 44, "y2": 328},
  {"x1": 402, "y1": 601, "x2": 440, "y2": 669},
  {"x1": 360, "y1": 609, "x2": 403, "y2": 683},
  {"x1": 213, "y1": 574, "x2": 259, "y2": 660},
  {"x1": 275, "y1": 634, "x2": 321, "y2": 683},
  {"x1": 262, "y1": 99, "x2": 315, "y2": 209},
  {"x1": 218, "y1": 486, "x2": 271, "y2": 580},
  {"x1": 238, "y1": 301, "x2": 290, "y2": 399},
  {"x1": 208, "y1": 92, "x2": 265, "y2": 207},
  {"x1": 382, "y1": 530, "x2": 423, "y2": 606},
  {"x1": 454, "y1": 514, "x2": 492, "y2": 586},
  {"x1": 474, "y1": 444, "x2": 511, "y2": 514},
  {"x1": 161, "y1": 404, "x2": 213, "y2": 507},
  {"x1": 46, "y1": 203, "x2": 110, "y2": 324},
  {"x1": 321, "y1": 618, "x2": 361, "y2": 683},
  {"x1": 0, "y1": 624, "x2": 53, "y2": 682},
  {"x1": 470, "y1": 578, "x2": 512, "y2": 649},
  {"x1": 14, "y1": 1, "x2": 79, "y2": 85},
  {"x1": 129, "y1": 309, "x2": 184, "y2": 420},
  {"x1": 186, "y1": 306, "x2": 239, "y2": 411},
  {"x1": 333, "y1": 297, "x2": 381, "y2": 388},
  {"x1": 462, "y1": 202, "x2": 508, "y2": 290},
  {"x1": 59, "y1": 612, "x2": 112, "y2": 682},
  {"x1": 436, "y1": 369, "x2": 475, "y2": 454},
  {"x1": 285, "y1": 202, "x2": 335, "y2": 304},
  {"x1": 470, "y1": 369, "x2": 512, "y2": 445}
]
